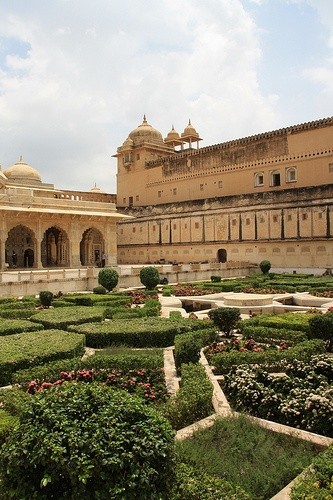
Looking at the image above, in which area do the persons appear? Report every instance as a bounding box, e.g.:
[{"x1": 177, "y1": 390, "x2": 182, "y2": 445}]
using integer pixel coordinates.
[
  {"x1": 12, "y1": 252, "x2": 17, "y2": 268},
  {"x1": 95, "y1": 251, "x2": 101, "y2": 268},
  {"x1": 101, "y1": 251, "x2": 108, "y2": 267}
]
